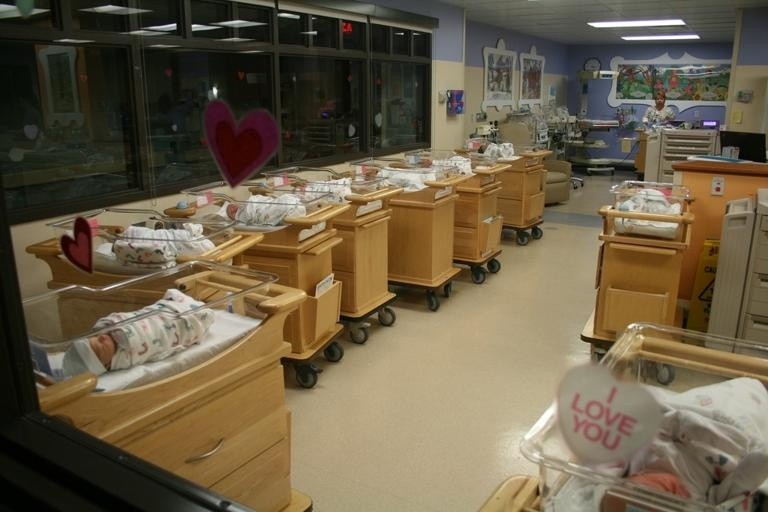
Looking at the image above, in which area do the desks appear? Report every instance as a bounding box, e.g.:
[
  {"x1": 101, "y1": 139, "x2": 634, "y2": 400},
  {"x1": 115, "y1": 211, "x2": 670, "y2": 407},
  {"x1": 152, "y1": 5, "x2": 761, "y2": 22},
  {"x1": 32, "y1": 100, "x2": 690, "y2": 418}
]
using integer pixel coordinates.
[{"x1": 668, "y1": 159, "x2": 767, "y2": 305}]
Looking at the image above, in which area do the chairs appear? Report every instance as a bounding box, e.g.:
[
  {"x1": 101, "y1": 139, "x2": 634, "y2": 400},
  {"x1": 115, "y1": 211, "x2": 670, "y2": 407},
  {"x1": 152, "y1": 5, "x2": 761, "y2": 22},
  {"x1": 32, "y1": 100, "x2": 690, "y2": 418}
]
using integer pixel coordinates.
[{"x1": 541, "y1": 159, "x2": 572, "y2": 210}]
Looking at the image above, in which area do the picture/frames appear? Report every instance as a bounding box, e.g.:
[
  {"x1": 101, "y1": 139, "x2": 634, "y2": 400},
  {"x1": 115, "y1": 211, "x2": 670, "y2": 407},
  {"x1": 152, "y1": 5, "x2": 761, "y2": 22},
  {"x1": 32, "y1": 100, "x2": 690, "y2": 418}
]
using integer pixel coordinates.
[
  {"x1": 479, "y1": 36, "x2": 517, "y2": 116},
  {"x1": 518, "y1": 44, "x2": 546, "y2": 111},
  {"x1": 608, "y1": 52, "x2": 732, "y2": 114}
]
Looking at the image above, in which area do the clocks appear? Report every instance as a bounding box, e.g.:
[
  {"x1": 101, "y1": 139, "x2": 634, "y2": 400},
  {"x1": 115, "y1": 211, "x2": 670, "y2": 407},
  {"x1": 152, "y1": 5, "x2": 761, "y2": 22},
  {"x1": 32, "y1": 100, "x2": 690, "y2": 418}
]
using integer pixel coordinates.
[{"x1": 584, "y1": 57, "x2": 601, "y2": 76}]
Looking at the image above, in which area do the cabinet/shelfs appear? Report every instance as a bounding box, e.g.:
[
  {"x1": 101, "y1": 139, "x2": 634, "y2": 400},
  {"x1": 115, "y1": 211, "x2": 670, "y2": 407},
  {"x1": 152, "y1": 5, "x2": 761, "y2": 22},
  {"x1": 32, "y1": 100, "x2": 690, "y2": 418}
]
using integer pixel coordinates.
[
  {"x1": 632, "y1": 127, "x2": 648, "y2": 182},
  {"x1": 473, "y1": 318, "x2": 768, "y2": 512}
]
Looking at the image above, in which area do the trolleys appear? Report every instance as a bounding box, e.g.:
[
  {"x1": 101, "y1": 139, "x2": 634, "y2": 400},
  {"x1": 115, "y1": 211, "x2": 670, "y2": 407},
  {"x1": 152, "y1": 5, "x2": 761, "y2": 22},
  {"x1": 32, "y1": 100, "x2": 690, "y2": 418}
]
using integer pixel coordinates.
[{"x1": 34, "y1": 257, "x2": 313, "y2": 512}]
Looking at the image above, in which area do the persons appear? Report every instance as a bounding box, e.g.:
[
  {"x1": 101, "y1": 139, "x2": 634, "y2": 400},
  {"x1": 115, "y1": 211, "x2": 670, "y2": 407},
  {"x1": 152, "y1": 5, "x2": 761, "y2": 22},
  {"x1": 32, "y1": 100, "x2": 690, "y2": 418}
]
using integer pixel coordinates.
[
  {"x1": 592, "y1": 430, "x2": 768, "y2": 512},
  {"x1": 477, "y1": 144, "x2": 489, "y2": 154},
  {"x1": 417, "y1": 158, "x2": 432, "y2": 169},
  {"x1": 364, "y1": 169, "x2": 379, "y2": 182},
  {"x1": 642, "y1": 91, "x2": 674, "y2": 128},
  {"x1": 615, "y1": 64, "x2": 731, "y2": 101},
  {"x1": 62, "y1": 329, "x2": 119, "y2": 378},
  {"x1": 102, "y1": 225, "x2": 126, "y2": 250},
  {"x1": 224, "y1": 202, "x2": 241, "y2": 221},
  {"x1": 294, "y1": 183, "x2": 306, "y2": 195}
]
